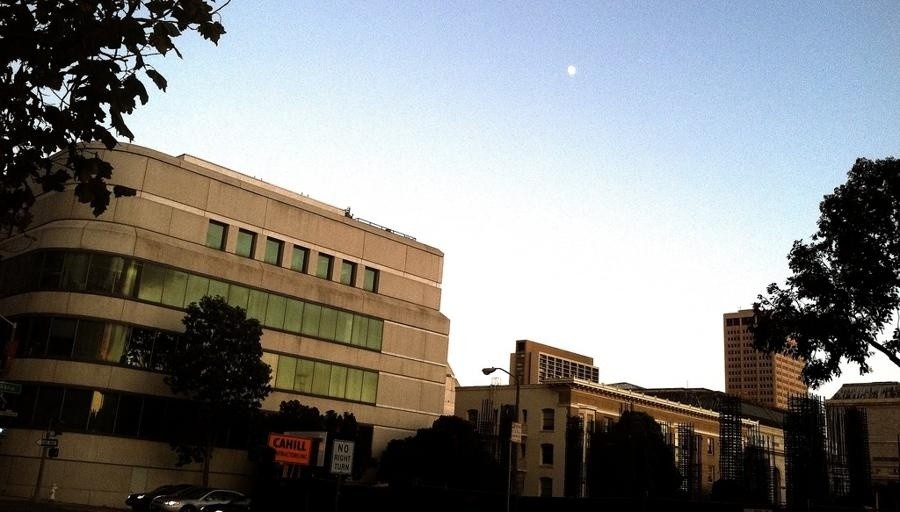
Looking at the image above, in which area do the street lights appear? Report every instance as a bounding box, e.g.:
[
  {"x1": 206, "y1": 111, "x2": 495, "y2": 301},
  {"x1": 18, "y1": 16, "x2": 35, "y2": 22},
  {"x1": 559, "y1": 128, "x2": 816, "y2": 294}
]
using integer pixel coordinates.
[{"x1": 481, "y1": 365, "x2": 518, "y2": 511}]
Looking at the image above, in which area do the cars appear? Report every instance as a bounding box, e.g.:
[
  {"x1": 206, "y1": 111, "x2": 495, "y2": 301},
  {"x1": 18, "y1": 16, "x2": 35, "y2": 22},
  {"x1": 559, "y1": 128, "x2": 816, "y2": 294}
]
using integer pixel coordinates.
[{"x1": 125, "y1": 483, "x2": 252, "y2": 511}]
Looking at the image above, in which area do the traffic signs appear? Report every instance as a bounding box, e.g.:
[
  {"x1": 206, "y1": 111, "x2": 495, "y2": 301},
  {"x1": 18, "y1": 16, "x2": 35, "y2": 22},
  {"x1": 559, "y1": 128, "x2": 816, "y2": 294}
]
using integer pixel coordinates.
[
  {"x1": 36, "y1": 438, "x2": 58, "y2": 447},
  {"x1": 0, "y1": 382, "x2": 21, "y2": 393}
]
